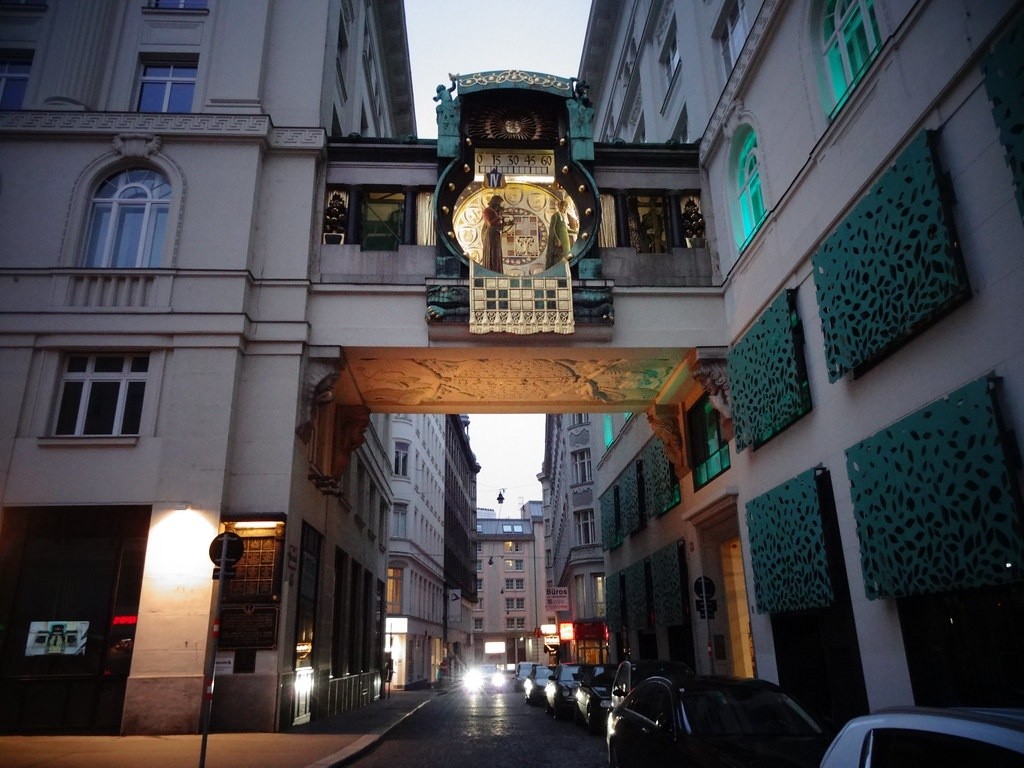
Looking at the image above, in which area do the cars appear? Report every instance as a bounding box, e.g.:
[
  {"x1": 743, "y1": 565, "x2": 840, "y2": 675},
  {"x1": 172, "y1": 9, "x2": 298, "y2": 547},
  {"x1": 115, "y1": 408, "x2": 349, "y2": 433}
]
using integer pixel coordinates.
[
  {"x1": 575, "y1": 665, "x2": 620, "y2": 735},
  {"x1": 545, "y1": 663, "x2": 597, "y2": 720},
  {"x1": 606, "y1": 676, "x2": 829, "y2": 768},
  {"x1": 818, "y1": 705, "x2": 1024, "y2": 768},
  {"x1": 515, "y1": 662, "x2": 542, "y2": 692},
  {"x1": 524, "y1": 666, "x2": 557, "y2": 706},
  {"x1": 470, "y1": 664, "x2": 501, "y2": 694},
  {"x1": 611, "y1": 660, "x2": 694, "y2": 708}
]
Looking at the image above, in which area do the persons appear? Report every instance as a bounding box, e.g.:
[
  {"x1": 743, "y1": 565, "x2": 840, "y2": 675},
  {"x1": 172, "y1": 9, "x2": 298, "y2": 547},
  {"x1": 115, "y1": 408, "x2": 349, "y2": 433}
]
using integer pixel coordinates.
[
  {"x1": 571, "y1": 77, "x2": 590, "y2": 108},
  {"x1": 689, "y1": 362, "x2": 728, "y2": 395},
  {"x1": 545, "y1": 200, "x2": 571, "y2": 270},
  {"x1": 439, "y1": 658, "x2": 447, "y2": 675},
  {"x1": 481, "y1": 195, "x2": 514, "y2": 274},
  {"x1": 340, "y1": 417, "x2": 367, "y2": 451},
  {"x1": 307, "y1": 362, "x2": 340, "y2": 405},
  {"x1": 647, "y1": 415, "x2": 679, "y2": 455},
  {"x1": 433, "y1": 81, "x2": 456, "y2": 103}
]
